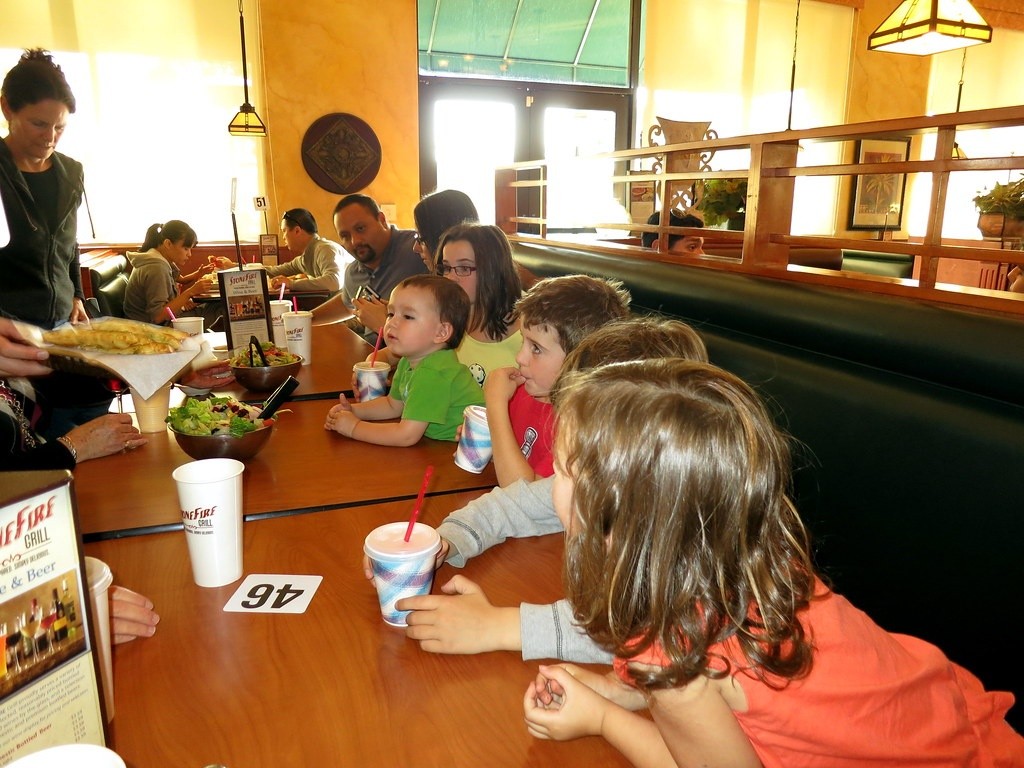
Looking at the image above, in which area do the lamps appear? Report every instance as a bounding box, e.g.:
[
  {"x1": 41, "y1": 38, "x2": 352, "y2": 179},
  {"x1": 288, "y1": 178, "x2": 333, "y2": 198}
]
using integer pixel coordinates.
[
  {"x1": 867, "y1": 1, "x2": 992, "y2": 56},
  {"x1": 950, "y1": 48, "x2": 969, "y2": 161},
  {"x1": 228, "y1": 1, "x2": 267, "y2": 137}
]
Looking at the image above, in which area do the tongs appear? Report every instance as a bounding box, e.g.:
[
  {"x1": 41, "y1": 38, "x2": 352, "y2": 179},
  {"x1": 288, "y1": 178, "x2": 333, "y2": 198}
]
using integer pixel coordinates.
[
  {"x1": 248, "y1": 335, "x2": 268, "y2": 367},
  {"x1": 211, "y1": 376, "x2": 300, "y2": 435}
]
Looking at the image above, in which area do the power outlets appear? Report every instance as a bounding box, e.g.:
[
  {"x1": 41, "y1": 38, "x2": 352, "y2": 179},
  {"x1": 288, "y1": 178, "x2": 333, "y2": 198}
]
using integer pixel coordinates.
[{"x1": 381, "y1": 204, "x2": 397, "y2": 221}]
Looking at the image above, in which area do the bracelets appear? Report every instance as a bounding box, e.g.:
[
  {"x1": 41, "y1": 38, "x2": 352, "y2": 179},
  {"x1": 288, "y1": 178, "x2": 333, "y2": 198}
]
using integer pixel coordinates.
[{"x1": 56, "y1": 436, "x2": 78, "y2": 461}]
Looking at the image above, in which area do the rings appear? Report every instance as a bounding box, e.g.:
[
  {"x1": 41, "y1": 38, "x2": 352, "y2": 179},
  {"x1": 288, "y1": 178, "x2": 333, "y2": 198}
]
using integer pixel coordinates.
[
  {"x1": 125, "y1": 441, "x2": 132, "y2": 449},
  {"x1": 277, "y1": 279, "x2": 280, "y2": 282}
]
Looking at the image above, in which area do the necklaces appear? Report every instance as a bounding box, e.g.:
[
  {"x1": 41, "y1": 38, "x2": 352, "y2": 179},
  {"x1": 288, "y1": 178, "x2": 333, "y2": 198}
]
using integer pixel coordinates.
[{"x1": 0, "y1": 380, "x2": 35, "y2": 449}]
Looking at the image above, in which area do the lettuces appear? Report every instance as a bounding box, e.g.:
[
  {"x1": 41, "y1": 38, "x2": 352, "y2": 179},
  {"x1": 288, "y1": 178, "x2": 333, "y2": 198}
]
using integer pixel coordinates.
[
  {"x1": 232, "y1": 342, "x2": 298, "y2": 367},
  {"x1": 163, "y1": 395, "x2": 258, "y2": 439}
]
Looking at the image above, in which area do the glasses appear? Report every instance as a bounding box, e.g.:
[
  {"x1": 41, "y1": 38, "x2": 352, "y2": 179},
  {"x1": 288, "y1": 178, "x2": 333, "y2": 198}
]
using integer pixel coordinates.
[
  {"x1": 411, "y1": 234, "x2": 426, "y2": 245},
  {"x1": 440, "y1": 265, "x2": 475, "y2": 277}
]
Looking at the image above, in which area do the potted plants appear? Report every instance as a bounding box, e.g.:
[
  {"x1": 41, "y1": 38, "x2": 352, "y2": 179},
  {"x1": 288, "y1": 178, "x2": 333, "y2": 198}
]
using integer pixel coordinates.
[
  {"x1": 695, "y1": 169, "x2": 748, "y2": 231},
  {"x1": 972, "y1": 173, "x2": 1024, "y2": 237}
]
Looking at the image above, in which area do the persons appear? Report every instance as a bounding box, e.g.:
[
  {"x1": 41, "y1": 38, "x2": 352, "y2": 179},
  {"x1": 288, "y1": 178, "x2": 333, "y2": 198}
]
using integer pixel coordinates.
[
  {"x1": 0, "y1": 48, "x2": 89, "y2": 376},
  {"x1": 123, "y1": 221, "x2": 216, "y2": 328},
  {"x1": 352, "y1": 224, "x2": 523, "y2": 404},
  {"x1": 308, "y1": 194, "x2": 431, "y2": 350},
  {"x1": 324, "y1": 274, "x2": 488, "y2": 442},
  {"x1": 413, "y1": 191, "x2": 480, "y2": 273},
  {"x1": 640, "y1": 211, "x2": 705, "y2": 257},
  {"x1": 524, "y1": 360, "x2": 1024, "y2": 768},
  {"x1": 107, "y1": 586, "x2": 159, "y2": 646},
  {"x1": 364, "y1": 319, "x2": 709, "y2": 663},
  {"x1": 0, "y1": 378, "x2": 148, "y2": 471},
  {"x1": 216, "y1": 208, "x2": 365, "y2": 334},
  {"x1": 455, "y1": 273, "x2": 630, "y2": 486}
]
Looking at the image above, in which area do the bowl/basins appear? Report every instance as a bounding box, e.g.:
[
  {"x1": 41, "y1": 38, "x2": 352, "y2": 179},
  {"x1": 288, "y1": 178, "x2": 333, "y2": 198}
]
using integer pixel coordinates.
[
  {"x1": 229, "y1": 354, "x2": 304, "y2": 393},
  {"x1": 167, "y1": 404, "x2": 272, "y2": 462},
  {"x1": 210, "y1": 284, "x2": 219, "y2": 289},
  {"x1": 174, "y1": 383, "x2": 211, "y2": 396}
]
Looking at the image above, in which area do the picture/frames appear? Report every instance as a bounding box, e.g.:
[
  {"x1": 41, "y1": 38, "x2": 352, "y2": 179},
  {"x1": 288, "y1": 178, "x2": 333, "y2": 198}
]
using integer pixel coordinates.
[{"x1": 847, "y1": 137, "x2": 912, "y2": 231}]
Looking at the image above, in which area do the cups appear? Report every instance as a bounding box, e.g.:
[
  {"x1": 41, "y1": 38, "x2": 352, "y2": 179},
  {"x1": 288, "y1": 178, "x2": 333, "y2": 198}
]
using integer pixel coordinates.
[
  {"x1": 85, "y1": 556, "x2": 115, "y2": 723},
  {"x1": 282, "y1": 311, "x2": 313, "y2": 365},
  {"x1": 246, "y1": 263, "x2": 262, "y2": 269},
  {"x1": 454, "y1": 405, "x2": 493, "y2": 473},
  {"x1": 363, "y1": 522, "x2": 442, "y2": 627},
  {"x1": 171, "y1": 317, "x2": 205, "y2": 337},
  {"x1": 3, "y1": 744, "x2": 126, "y2": 768},
  {"x1": 172, "y1": 458, "x2": 245, "y2": 587},
  {"x1": 269, "y1": 300, "x2": 293, "y2": 348},
  {"x1": 353, "y1": 361, "x2": 391, "y2": 403},
  {"x1": 128, "y1": 381, "x2": 172, "y2": 433}
]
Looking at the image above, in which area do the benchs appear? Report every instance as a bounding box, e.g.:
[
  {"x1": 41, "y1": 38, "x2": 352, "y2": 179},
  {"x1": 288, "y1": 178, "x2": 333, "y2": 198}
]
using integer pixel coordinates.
[
  {"x1": 79, "y1": 248, "x2": 130, "y2": 318},
  {"x1": 842, "y1": 248, "x2": 915, "y2": 278},
  {"x1": 508, "y1": 240, "x2": 1023, "y2": 737}
]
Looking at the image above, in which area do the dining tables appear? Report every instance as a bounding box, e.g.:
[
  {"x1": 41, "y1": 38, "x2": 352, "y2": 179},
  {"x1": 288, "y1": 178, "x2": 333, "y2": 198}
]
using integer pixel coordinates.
[
  {"x1": 191, "y1": 288, "x2": 331, "y2": 303},
  {"x1": 71, "y1": 289, "x2": 655, "y2": 768}
]
